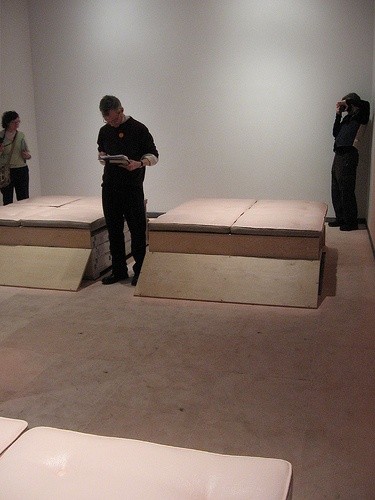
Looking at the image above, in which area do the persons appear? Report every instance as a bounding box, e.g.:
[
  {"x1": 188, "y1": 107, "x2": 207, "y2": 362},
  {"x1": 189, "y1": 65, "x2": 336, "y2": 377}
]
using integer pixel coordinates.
[
  {"x1": 0, "y1": 110, "x2": 32, "y2": 205},
  {"x1": 97, "y1": 95, "x2": 160, "y2": 286},
  {"x1": 328, "y1": 93, "x2": 370, "y2": 232}
]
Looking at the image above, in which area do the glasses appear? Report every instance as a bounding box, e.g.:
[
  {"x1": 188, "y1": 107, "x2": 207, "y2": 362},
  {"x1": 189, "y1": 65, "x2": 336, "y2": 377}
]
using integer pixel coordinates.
[{"x1": 102, "y1": 116, "x2": 120, "y2": 124}]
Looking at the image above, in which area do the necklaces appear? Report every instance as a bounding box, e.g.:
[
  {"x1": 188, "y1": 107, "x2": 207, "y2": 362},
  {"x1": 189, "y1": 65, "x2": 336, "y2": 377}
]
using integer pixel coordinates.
[{"x1": 2, "y1": 129, "x2": 18, "y2": 146}]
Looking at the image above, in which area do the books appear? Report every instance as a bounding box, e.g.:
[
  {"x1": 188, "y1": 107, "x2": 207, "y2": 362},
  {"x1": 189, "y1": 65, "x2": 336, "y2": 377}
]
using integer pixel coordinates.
[{"x1": 99, "y1": 155, "x2": 131, "y2": 167}]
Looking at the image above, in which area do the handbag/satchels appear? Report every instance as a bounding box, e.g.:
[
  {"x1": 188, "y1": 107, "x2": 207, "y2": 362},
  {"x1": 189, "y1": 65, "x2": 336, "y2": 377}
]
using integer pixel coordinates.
[{"x1": 0, "y1": 164, "x2": 10, "y2": 188}]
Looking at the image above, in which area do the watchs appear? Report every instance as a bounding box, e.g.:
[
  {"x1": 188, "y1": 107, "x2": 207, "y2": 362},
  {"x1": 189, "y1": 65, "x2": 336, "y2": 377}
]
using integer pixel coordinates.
[{"x1": 139, "y1": 160, "x2": 144, "y2": 170}]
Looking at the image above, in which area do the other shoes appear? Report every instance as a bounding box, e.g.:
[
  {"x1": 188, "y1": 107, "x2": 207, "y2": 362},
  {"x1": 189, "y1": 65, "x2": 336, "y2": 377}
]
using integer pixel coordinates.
[
  {"x1": 328, "y1": 219, "x2": 341, "y2": 227},
  {"x1": 130, "y1": 274, "x2": 139, "y2": 286},
  {"x1": 340, "y1": 223, "x2": 358, "y2": 231},
  {"x1": 101, "y1": 273, "x2": 129, "y2": 284}
]
